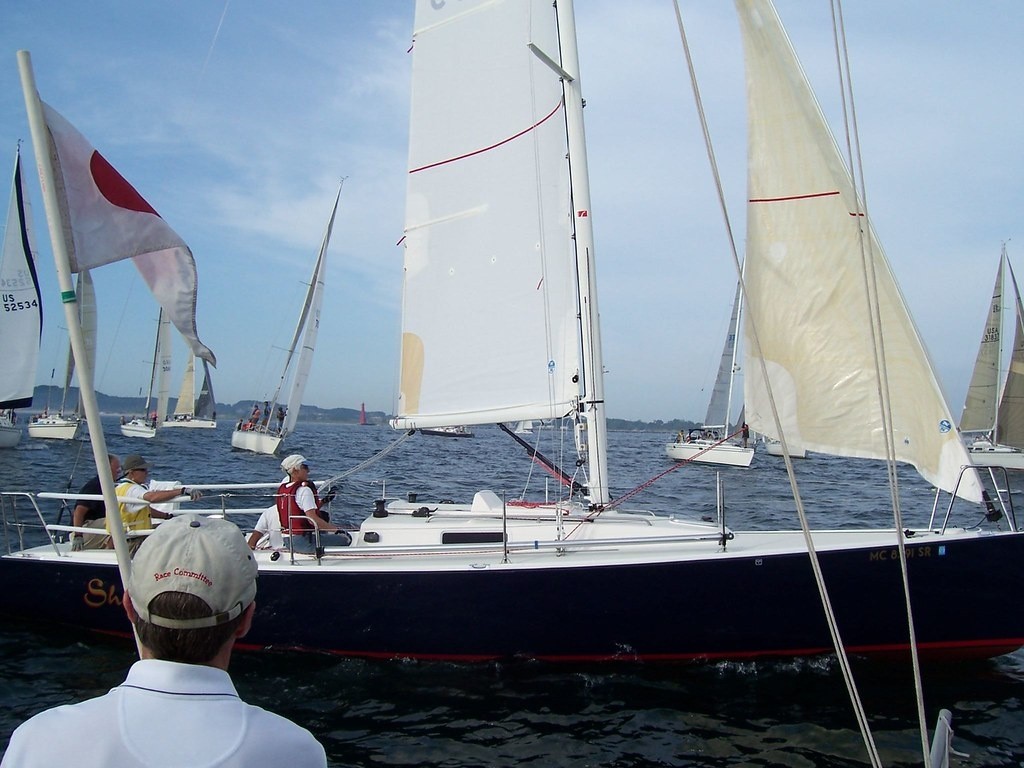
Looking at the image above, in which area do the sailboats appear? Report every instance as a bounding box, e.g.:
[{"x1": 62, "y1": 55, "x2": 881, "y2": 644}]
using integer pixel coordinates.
[
  {"x1": 162, "y1": 349, "x2": 218, "y2": 429},
  {"x1": 118, "y1": 305, "x2": 174, "y2": 438},
  {"x1": 0, "y1": 137, "x2": 44, "y2": 450},
  {"x1": 26, "y1": 270, "x2": 99, "y2": 440},
  {"x1": 511, "y1": 421, "x2": 536, "y2": 435},
  {"x1": 233, "y1": 174, "x2": 348, "y2": 457},
  {"x1": 0, "y1": 0, "x2": 1024, "y2": 680},
  {"x1": 663, "y1": 254, "x2": 757, "y2": 468},
  {"x1": 959, "y1": 238, "x2": 1024, "y2": 470}
]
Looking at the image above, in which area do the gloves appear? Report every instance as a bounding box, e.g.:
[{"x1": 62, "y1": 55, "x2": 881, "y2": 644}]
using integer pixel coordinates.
[
  {"x1": 71, "y1": 536, "x2": 85, "y2": 551},
  {"x1": 184, "y1": 488, "x2": 203, "y2": 501}
]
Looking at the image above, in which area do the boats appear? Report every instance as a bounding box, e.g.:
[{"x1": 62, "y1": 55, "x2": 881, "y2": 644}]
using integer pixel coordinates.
[
  {"x1": 762, "y1": 437, "x2": 807, "y2": 460},
  {"x1": 417, "y1": 426, "x2": 476, "y2": 439}
]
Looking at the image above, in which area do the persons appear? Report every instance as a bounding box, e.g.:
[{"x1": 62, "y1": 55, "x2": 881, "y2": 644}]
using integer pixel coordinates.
[
  {"x1": 131, "y1": 415, "x2": 135, "y2": 421},
  {"x1": 6, "y1": 409, "x2": 16, "y2": 426},
  {"x1": 241, "y1": 401, "x2": 262, "y2": 432},
  {"x1": 0, "y1": 514, "x2": 328, "y2": 768},
  {"x1": 235, "y1": 418, "x2": 245, "y2": 431},
  {"x1": 677, "y1": 430, "x2": 685, "y2": 443},
  {"x1": 113, "y1": 451, "x2": 203, "y2": 560},
  {"x1": 276, "y1": 407, "x2": 285, "y2": 435},
  {"x1": 72, "y1": 450, "x2": 121, "y2": 552},
  {"x1": 740, "y1": 422, "x2": 750, "y2": 448},
  {"x1": 261, "y1": 401, "x2": 272, "y2": 434},
  {"x1": 248, "y1": 502, "x2": 294, "y2": 552},
  {"x1": 119, "y1": 416, "x2": 126, "y2": 425},
  {"x1": 276, "y1": 453, "x2": 349, "y2": 553},
  {"x1": 150, "y1": 408, "x2": 157, "y2": 429}
]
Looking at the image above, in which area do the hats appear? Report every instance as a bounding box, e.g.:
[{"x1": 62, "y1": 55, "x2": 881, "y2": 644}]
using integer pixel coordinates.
[
  {"x1": 123, "y1": 455, "x2": 151, "y2": 470},
  {"x1": 281, "y1": 454, "x2": 312, "y2": 470},
  {"x1": 128, "y1": 514, "x2": 258, "y2": 629}
]
